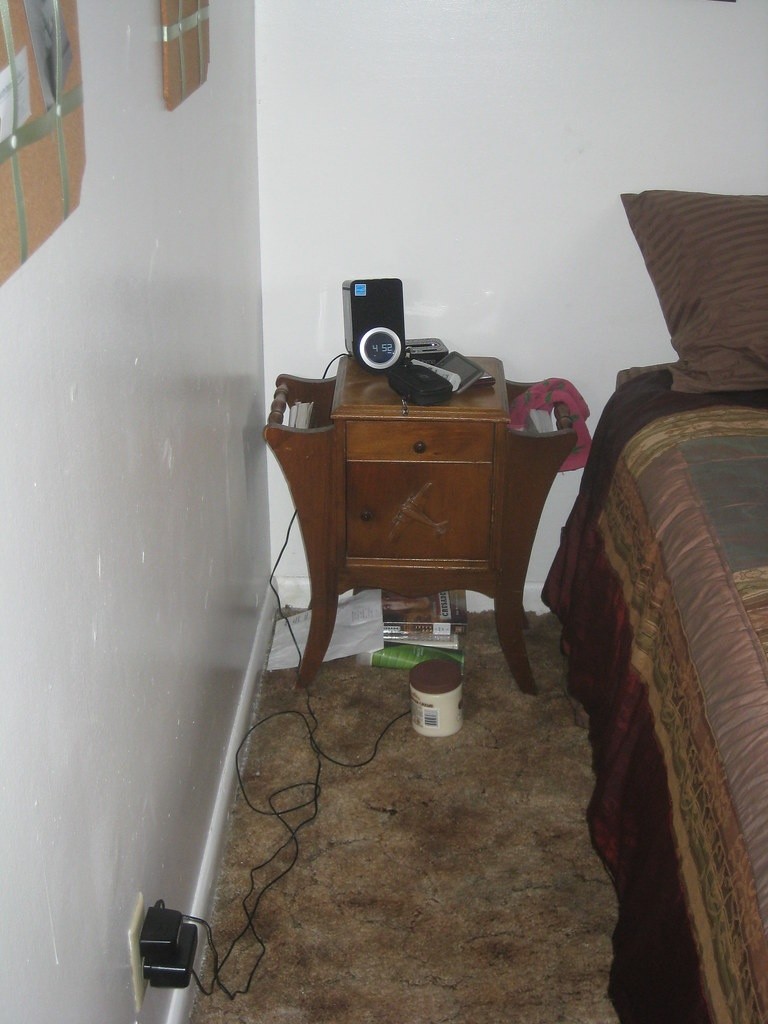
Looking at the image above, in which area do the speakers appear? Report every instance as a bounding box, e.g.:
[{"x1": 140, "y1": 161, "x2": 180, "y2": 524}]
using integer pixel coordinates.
[{"x1": 342, "y1": 278, "x2": 405, "y2": 376}]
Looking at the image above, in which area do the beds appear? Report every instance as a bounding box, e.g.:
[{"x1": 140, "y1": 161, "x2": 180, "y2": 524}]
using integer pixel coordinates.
[{"x1": 541, "y1": 360, "x2": 768, "y2": 1024}]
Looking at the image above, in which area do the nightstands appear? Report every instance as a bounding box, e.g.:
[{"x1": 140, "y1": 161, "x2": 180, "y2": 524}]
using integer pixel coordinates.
[{"x1": 264, "y1": 355, "x2": 579, "y2": 695}]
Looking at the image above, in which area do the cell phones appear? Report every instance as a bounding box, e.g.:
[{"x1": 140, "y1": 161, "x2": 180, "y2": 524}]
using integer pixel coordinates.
[{"x1": 435, "y1": 351, "x2": 484, "y2": 394}]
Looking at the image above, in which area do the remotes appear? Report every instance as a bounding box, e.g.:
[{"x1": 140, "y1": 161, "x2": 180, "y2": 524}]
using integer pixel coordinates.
[{"x1": 411, "y1": 359, "x2": 461, "y2": 392}]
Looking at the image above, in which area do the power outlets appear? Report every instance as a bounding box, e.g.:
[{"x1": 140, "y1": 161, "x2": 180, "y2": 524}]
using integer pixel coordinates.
[{"x1": 129, "y1": 889, "x2": 150, "y2": 1010}]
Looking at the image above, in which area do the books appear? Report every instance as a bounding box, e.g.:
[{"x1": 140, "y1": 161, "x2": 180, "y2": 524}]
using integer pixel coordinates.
[
  {"x1": 525, "y1": 408, "x2": 554, "y2": 433},
  {"x1": 353, "y1": 586, "x2": 469, "y2": 636},
  {"x1": 294, "y1": 401, "x2": 318, "y2": 429}
]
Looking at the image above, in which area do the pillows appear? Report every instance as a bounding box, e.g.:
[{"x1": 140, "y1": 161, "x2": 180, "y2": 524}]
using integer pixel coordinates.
[{"x1": 622, "y1": 188, "x2": 768, "y2": 393}]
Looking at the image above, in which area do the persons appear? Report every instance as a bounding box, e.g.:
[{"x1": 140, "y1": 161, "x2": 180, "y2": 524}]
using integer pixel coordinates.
[{"x1": 381, "y1": 592, "x2": 440, "y2": 615}]
